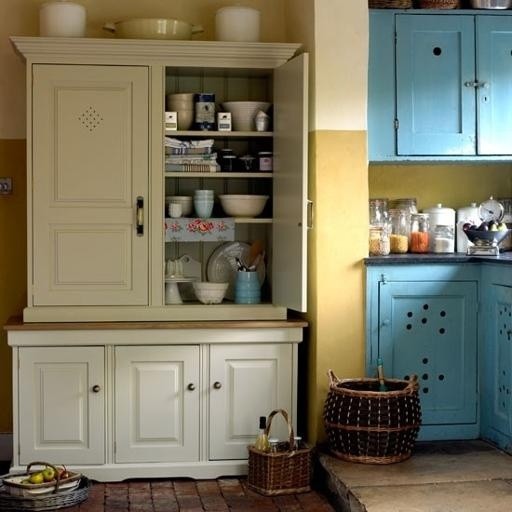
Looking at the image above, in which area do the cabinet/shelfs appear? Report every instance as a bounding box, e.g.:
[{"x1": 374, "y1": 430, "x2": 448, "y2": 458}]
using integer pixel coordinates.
[
  {"x1": 490, "y1": 284, "x2": 511, "y2": 436},
  {"x1": 17, "y1": 346, "x2": 106, "y2": 468},
  {"x1": 394, "y1": 13, "x2": 511, "y2": 155},
  {"x1": 378, "y1": 278, "x2": 479, "y2": 426},
  {"x1": 7, "y1": 35, "x2": 314, "y2": 322},
  {"x1": 114, "y1": 344, "x2": 292, "y2": 463}
]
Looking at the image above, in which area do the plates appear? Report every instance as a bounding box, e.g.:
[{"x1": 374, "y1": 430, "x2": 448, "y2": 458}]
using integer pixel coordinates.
[{"x1": 206, "y1": 241, "x2": 266, "y2": 300}]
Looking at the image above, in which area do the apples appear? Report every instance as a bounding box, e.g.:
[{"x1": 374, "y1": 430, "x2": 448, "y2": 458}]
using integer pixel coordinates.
[{"x1": 20, "y1": 467, "x2": 68, "y2": 484}]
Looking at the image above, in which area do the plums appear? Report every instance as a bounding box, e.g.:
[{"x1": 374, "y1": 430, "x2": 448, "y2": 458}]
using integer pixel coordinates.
[{"x1": 463, "y1": 220, "x2": 507, "y2": 231}]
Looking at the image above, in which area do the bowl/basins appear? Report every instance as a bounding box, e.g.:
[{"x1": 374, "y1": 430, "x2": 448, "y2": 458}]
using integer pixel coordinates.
[
  {"x1": 168, "y1": 93, "x2": 194, "y2": 130},
  {"x1": 217, "y1": 194, "x2": 270, "y2": 217},
  {"x1": 165, "y1": 189, "x2": 215, "y2": 218},
  {"x1": 192, "y1": 281, "x2": 229, "y2": 304}
]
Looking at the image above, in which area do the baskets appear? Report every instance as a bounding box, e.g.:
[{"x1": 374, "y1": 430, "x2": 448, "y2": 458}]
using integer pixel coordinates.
[
  {"x1": 0, "y1": 468, "x2": 88, "y2": 510},
  {"x1": 245, "y1": 406, "x2": 314, "y2": 496},
  {"x1": 2, "y1": 460, "x2": 82, "y2": 496},
  {"x1": 320, "y1": 365, "x2": 423, "y2": 467}
]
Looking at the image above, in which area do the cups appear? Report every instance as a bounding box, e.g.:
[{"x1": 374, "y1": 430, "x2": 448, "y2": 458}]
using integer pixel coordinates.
[{"x1": 235, "y1": 271, "x2": 261, "y2": 304}]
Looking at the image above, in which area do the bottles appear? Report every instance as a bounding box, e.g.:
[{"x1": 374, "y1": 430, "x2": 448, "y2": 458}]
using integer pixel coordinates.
[
  {"x1": 254, "y1": 416, "x2": 272, "y2": 454},
  {"x1": 376, "y1": 357, "x2": 389, "y2": 391},
  {"x1": 195, "y1": 93, "x2": 217, "y2": 131},
  {"x1": 369, "y1": 198, "x2": 483, "y2": 257}
]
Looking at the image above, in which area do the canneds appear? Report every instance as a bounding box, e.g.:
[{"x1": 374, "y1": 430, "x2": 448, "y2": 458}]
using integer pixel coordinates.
[
  {"x1": 221, "y1": 148, "x2": 236, "y2": 171},
  {"x1": 430, "y1": 225, "x2": 455, "y2": 253},
  {"x1": 369, "y1": 198, "x2": 430, "y2": 256},
  {"x1": 258, "y1": 151, "x2": 273, "y2": 172}
]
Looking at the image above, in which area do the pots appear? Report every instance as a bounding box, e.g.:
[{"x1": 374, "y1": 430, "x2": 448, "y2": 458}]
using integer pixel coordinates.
[
  {"x1": 39, "y1": 1, "x2": 87, "y2": 37},
  {"x1": 102, "y1": 17, "x2": 205, "y2": 41},
  {"x1": 213, "y1": 2, "x2": 263, "y2": 42}
]
